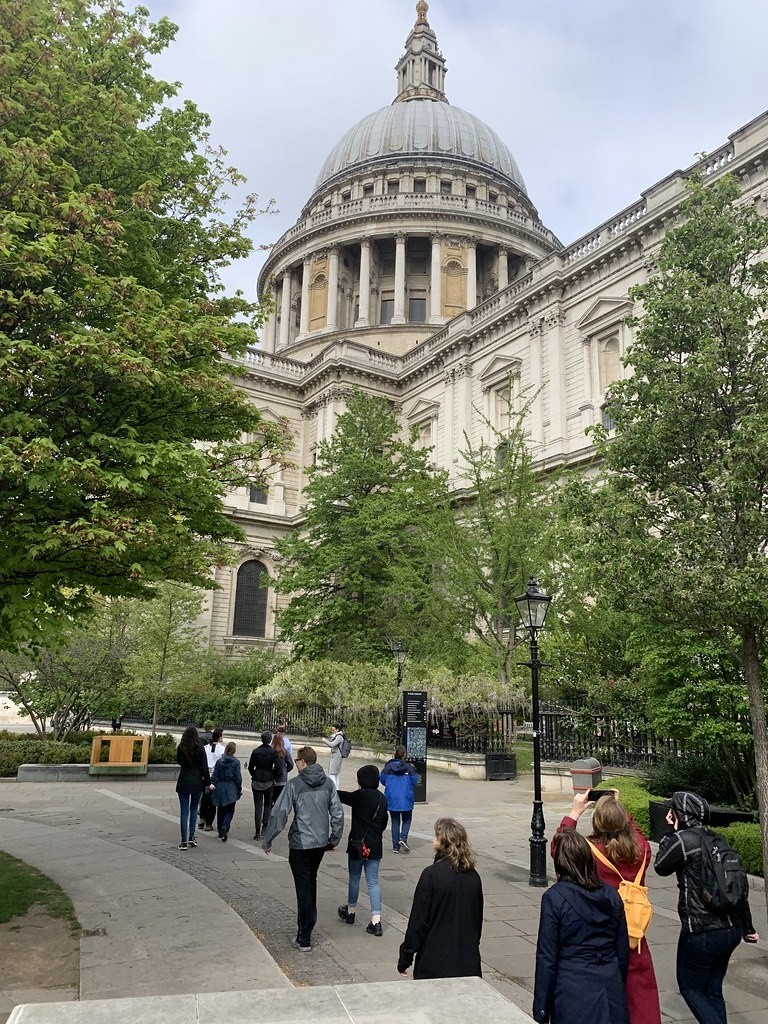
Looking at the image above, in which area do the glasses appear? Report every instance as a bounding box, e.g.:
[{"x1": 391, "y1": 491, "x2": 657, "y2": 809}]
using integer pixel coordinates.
[{"x1": 294, "y1": 759, "x2": 305, "y2": 763}]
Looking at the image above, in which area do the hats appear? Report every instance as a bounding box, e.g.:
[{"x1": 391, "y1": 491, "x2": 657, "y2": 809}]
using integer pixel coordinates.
[{"x1": 204, "y1": 720, "x2": 215, "y2": 727}]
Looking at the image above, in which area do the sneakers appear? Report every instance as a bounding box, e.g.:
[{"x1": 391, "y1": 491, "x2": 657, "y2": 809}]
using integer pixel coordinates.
[
  {"x1": 366, "y1": 921, "x2": 382, "y2": 936},
  {"x1": 392, "y1": 847, "x2": 399, "y2": 854},
  {"x1": 399, "y1": 837, "x2": 411, "y2": 853},
  {"x1": 291, "y1": 937, "x2": 311, "y2": 951},
  {"x1": 188, "y1": 837, "x2": 198, "y2": 846},
  {"x1": 338, "y1": 904, "x2": 356, "y2": 924},
  {"x1": 178, "y1": 843, "x2": 187, "y2": 849}
]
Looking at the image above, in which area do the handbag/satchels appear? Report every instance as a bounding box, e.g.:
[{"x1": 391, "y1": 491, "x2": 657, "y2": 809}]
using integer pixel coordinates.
[{"x1": 348, "y1": 840, "x2": 369, "y2": 860}]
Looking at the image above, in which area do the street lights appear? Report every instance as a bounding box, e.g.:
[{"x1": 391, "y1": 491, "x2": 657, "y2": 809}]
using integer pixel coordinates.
[
  {"x1": 392, "y1": 638, "x2": 409, "y2": 748},
  {"x1": 512, "y1": 573, "x2": 554, "y2": 888}
]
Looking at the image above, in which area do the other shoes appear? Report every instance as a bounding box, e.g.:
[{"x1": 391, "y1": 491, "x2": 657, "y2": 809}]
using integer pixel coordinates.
[
  {"x1": 198, "y1": 818, "x2": 204, "y2": 828},
  {"x1": 260, "y1": 832, "x2": 264, "y2": 839},
  {"x1": 205, "y1": 826, "x2": 213, "y2": 830},
  {"x1": 220, "y1": 827, "x2": 228, "y2": 843},
  {"x1": 254, "y1": 835, "x2": 261, "y2": 840}
]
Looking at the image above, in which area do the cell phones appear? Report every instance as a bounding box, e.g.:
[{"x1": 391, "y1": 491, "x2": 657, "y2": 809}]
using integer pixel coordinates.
[{"x1": 587, "y1": 790, "x2": 614, "y2": 801}]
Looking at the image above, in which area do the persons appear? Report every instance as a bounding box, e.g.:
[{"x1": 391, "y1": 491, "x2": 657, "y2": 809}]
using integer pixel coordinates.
[
  {"x1": 248, "y1": 731, "x2": 283, "y2": 841},
  {"x1": 397, "y1": 818, "x2": 484, "y2": 980},
  {"x1": 211, "y1": 742, "x2": 242, "y2": 843},
  {"x1": 261, "y1": 747, "x2": 344, "y2": 951},
  {"x1": 548, "y1": 789, "x2": 660, "y2": 1024},
  {"x1": 175, "y1": 726, "x2": 215, "y2": 850},
  {"x1": 271, "y1": 736, "x2": 294, "y2": 808},
  {"x1": 197, "y1": 729, "x2": 225, "y2": 830},
  {"x1": 532, "y1": 829, "x2": 630, "y2": 1024},
  {"x1": 337, "y1": 765, "x2": 389, "y2": 936},
  {"x1": 322, "y1": 724, "x2": 344, "y2": 790},
  {"x1": 199, "y1": 720, "x2": 214, "y2": 746},
  {"x1": 111, "y1": 716, "x2": 122, "y2": 732},
  {"x1": 269, "y1": 727, "x2": 293, "y2": 754},
  {"x1": 380, "y1": 745, "x2": 419, "y2": 852},
  {"x1": 654, "y1": 792, "x2": 759, "y2": 1024}
]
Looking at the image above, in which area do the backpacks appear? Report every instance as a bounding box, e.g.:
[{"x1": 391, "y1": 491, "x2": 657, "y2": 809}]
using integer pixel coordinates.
[
  {"x1": 334, "y1": 734, "x2": 351, "y2": 758},
  {"x1": 690, "y1": 827, "x2": 749, "y2": 916},
  {"x1": 585, "y1": 839, "x2": 654, "y2": 954}
]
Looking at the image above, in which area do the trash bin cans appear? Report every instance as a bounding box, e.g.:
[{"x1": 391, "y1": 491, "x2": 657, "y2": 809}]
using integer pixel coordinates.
[
  {"x1": 570, "y1": 757, "x2": 603, "y2": 795},
  {"x1": 486, "y1": 754, "x2": 516, "y2": 781}
]
